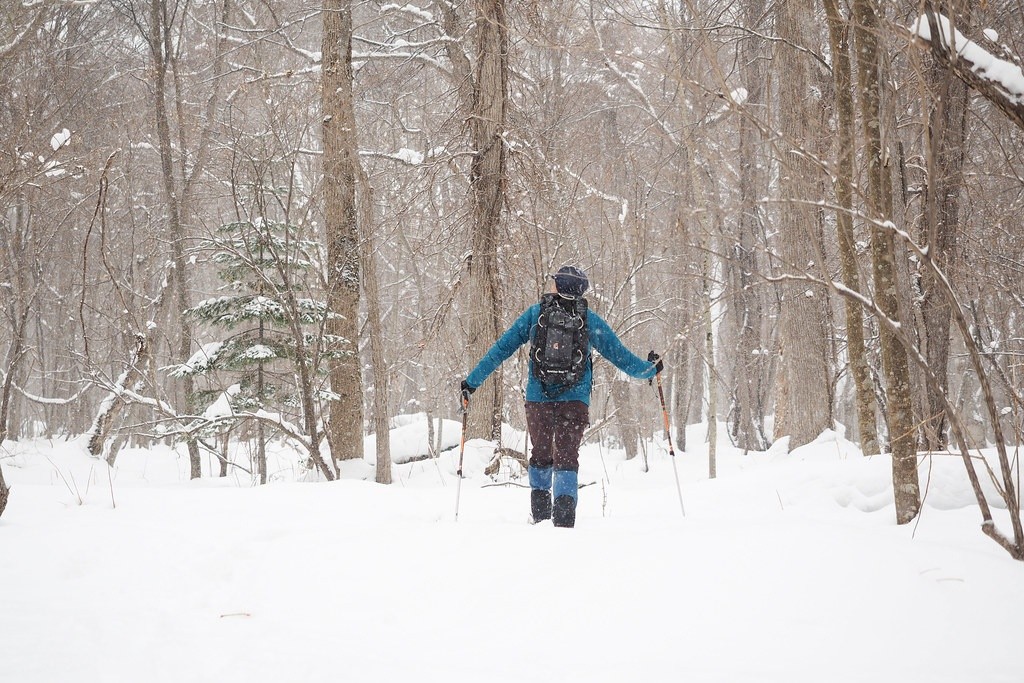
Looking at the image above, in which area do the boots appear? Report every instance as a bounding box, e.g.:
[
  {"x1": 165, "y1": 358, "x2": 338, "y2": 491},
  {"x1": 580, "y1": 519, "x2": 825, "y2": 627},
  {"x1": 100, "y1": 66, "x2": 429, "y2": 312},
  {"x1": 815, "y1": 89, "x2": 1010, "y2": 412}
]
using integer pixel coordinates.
[
  {"x1": 551, "y1": 465, "x2": 576, "y2": 527},
  {"x1": 529, "y1": 458, "x2": 552, "y2": 522}
]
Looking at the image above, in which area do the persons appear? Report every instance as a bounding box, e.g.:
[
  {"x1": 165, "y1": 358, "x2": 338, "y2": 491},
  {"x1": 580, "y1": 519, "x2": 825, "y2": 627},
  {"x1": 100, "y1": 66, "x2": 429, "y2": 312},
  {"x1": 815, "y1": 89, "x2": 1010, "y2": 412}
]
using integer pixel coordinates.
[{"x1": 461, "y1": 266, "x2": 664, "y2": 529}]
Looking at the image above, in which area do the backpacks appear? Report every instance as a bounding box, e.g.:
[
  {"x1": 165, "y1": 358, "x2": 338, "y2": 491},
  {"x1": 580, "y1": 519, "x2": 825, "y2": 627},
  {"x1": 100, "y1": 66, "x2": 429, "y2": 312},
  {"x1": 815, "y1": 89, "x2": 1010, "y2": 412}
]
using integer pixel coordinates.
[{"x1": 529, "y1": 292, "x2": 589, "y2": 400}]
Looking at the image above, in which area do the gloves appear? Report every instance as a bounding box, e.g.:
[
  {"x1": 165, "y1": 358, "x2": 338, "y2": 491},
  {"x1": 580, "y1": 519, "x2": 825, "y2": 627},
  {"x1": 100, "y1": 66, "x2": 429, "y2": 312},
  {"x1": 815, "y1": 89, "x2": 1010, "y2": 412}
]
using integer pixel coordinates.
[
  {"x1": 461, "y1": 380, "x2": 476, "y2": 401},
  {"x1": 648, "y1": 350, "x2": 664, "y2": 374}
]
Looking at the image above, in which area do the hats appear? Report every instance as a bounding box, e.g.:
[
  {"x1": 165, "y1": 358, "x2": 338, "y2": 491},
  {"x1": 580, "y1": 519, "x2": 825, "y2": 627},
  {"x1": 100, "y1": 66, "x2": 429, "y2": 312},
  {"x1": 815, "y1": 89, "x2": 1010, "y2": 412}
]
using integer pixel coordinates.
[{"x1": 551, "y1": 265, "x2": 590, "y2": 296}]
